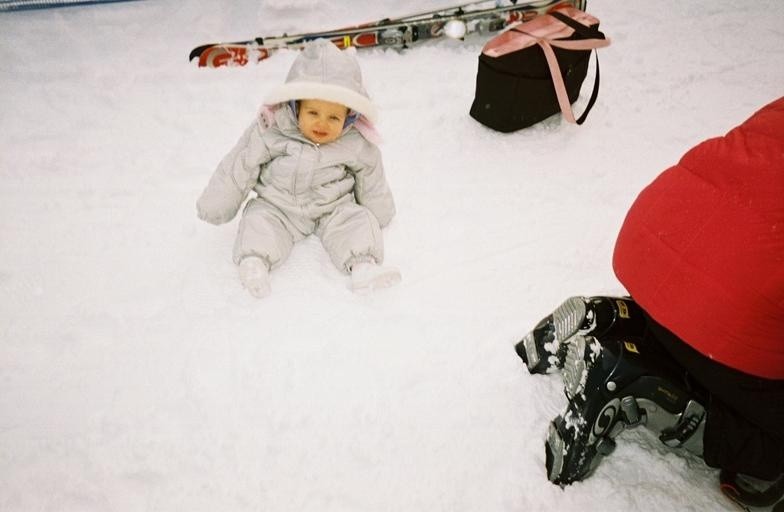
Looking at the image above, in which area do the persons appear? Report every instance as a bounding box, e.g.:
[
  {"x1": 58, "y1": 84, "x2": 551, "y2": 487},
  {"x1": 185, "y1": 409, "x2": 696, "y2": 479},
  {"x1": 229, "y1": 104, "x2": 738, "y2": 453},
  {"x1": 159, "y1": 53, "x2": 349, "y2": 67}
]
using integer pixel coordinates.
[
  {"x1": 192, "y1": 37, "x2": 400, "y2": 304},
  {"x1": 515, "y1": 95, "x2": 784, "y2": 507}
]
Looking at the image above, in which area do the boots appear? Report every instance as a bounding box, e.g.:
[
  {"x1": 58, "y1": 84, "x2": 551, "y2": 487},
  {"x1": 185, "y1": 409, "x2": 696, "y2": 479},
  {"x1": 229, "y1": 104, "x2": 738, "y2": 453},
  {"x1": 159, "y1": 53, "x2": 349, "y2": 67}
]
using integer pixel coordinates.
[
  {"x1": 546, "y1": 335, "x2": 703, "y2": 486},
  {"x1": 517, "y1": 295, "x2": 640, "y2": 375}
]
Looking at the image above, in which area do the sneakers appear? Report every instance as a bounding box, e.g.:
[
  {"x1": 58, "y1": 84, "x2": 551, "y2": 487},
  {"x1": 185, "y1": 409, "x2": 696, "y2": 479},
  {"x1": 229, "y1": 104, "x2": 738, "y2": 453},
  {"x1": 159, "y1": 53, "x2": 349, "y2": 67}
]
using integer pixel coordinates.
[
  {"x1": 238, "y1": 259, "x2": 270, "y2": 297},
  {"x1": 351, "y1": 262, "x2": 403, "y2": 297}
]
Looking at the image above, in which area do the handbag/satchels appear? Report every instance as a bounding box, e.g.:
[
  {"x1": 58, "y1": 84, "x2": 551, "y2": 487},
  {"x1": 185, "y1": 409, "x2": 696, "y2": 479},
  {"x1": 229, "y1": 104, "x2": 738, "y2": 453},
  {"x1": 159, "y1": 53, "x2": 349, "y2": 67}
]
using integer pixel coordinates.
[{"x1": 470, "y1": 7, "x2": 600, "y2": 133}]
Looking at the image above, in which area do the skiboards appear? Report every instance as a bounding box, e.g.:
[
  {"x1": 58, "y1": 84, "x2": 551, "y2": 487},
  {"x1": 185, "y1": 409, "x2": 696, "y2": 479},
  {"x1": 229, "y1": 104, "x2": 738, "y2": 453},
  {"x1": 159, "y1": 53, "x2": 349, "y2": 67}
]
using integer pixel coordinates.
[{"x1": 189, "y1": 0, "x2": 587, "y2": 69}]
